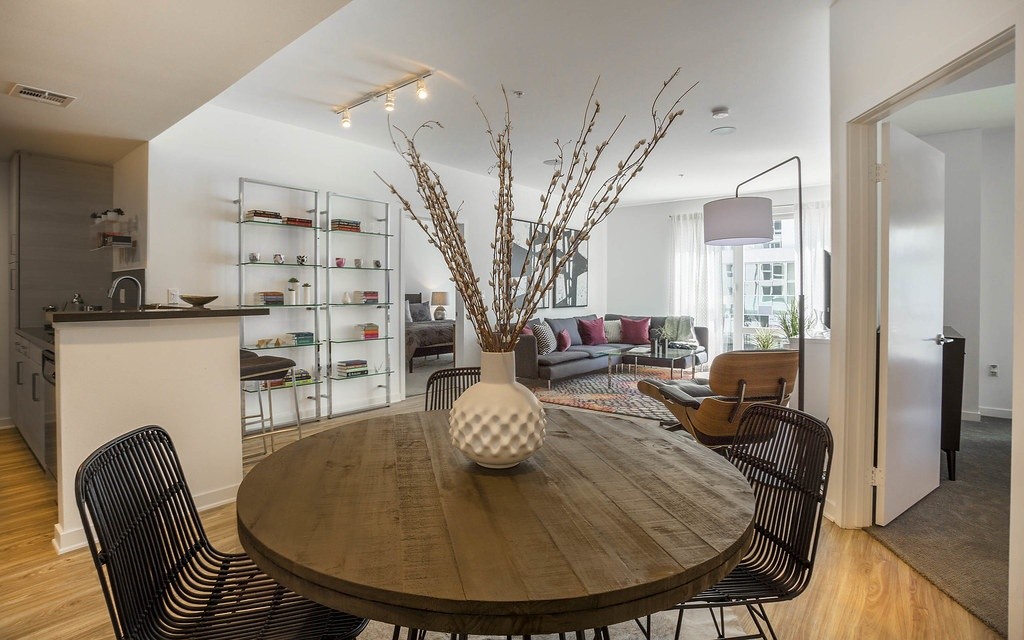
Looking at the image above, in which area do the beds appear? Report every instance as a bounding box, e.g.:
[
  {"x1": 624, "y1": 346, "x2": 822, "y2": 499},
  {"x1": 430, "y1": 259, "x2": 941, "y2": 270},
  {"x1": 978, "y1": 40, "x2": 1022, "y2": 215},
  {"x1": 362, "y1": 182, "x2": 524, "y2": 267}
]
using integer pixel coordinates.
[{"x1": 405, "y1": 293, "x2": 455, "y2": 374}]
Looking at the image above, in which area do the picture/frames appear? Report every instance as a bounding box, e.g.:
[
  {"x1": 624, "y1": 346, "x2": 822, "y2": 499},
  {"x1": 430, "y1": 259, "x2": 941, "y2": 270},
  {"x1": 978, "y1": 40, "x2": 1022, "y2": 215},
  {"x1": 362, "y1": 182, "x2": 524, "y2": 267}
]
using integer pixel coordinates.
[{"x1": 507, "y1": 218, "x2": 588, "y2": 309}]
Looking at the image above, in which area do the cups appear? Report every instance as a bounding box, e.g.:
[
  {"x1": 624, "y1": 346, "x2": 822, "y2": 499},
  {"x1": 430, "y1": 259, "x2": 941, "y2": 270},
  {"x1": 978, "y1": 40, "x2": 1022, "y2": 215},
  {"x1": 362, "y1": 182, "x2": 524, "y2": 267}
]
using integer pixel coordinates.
[
  {"x1": 651, "y1": 338, "x2": 658, "y2": 354},
  {"x1": 296, "y1": 255, "x2": 307, "y2": 264},
  {"x1": 336, "y1": 258, "x2": 346, "y2": 268},
  {"x1": 373, "y1": 259, "x2": 382, "y2": 268},
  {"x1": 249, "y1": 252, "x2": 261, "y2": 263},
  {"x1": 354, "y1": 259, "x2": 363, "y2": 268},
  {"x1": 84, "y1": 305, "x2": 103, "y2": 312},
  {"x1": 273, "y1": 253, "x2": 285, "y2": 264}
]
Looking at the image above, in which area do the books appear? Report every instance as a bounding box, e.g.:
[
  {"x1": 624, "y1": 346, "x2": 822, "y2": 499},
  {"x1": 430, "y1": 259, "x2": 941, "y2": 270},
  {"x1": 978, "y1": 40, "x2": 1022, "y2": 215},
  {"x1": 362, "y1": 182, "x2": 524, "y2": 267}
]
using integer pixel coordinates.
[
  {"x1": 96, "y1": 231, "x2": 133, "y2": 248},
  {"x1": 243, "y1": 209, "x2": 283, "y2": 225},
  {"x1": 255, "y1": 291, "x2": 286, "y2": 305},
  {"x1": 285, "y1": 332, "x2": 314, "y2": 346},
  {"x1": 338, "y1": 359, "x2": 369, "y2": 377},
  {"x1": 356, "y1": 290, "x2": 379, "y2": 305},
  {"x1": 357, "y1": 323, "x2": 379, "y2": 340},
  {"x1": 262, "y1": 368, "x2": 313, "y2": 387},
  {"x1": 284, "y1": 217, "x2": 313, "y2": 227},
  {"x1": 331, "y1": 219, "x2": 361, "y2": 233}
]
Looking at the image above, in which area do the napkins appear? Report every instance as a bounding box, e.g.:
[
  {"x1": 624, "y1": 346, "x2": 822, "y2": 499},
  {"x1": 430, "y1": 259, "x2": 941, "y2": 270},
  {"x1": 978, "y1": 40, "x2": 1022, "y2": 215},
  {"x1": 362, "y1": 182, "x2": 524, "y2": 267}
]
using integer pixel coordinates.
[{"x1": 240, "y1": 350, "x2": 301, "y2": 465}]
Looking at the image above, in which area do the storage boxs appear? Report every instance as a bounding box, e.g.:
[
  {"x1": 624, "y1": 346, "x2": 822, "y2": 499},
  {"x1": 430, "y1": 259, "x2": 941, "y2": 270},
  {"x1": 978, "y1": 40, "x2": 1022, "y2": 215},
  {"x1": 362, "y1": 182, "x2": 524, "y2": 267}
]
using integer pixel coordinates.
[{"x1": 90, "y1": 208, "x2": 125, "y2": 224}]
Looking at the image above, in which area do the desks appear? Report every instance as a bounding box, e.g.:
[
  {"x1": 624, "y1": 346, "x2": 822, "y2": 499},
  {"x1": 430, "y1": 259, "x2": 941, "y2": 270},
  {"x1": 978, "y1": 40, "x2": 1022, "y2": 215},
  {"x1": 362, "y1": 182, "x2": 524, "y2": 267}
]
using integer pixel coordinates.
[
  {"x1": 237, "y1": 409, "x2": 756, "y2": 640},
  {"x1": 876, "y1": 325, "x2": 966, "y2": 481}
]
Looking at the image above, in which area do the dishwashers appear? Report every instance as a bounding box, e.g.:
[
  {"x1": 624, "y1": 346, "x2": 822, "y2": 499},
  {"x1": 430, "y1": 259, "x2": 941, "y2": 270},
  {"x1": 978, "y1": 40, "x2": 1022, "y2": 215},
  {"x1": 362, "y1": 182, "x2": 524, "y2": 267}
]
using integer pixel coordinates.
[{"x1": 40, "y1": 349, "x2": 57, "y2": 488}]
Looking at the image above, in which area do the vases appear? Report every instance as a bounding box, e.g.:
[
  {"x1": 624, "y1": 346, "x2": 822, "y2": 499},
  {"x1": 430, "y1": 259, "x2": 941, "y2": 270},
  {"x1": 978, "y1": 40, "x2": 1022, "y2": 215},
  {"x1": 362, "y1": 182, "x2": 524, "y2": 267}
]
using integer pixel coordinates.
[{"x1": 448, "y1": 350, "x2": 546, "y2": 468}]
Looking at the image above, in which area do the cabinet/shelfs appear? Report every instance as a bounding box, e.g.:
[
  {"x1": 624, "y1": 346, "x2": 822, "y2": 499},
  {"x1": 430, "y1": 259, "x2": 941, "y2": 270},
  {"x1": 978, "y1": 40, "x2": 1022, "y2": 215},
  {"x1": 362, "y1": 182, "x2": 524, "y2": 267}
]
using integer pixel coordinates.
[
  {"x1": 89, "y1": 220, "x2": 134, "y2": 252},
  {"x1": 13, "y1": 350, "x2": 45, "y2": 468},
  {"x1": 233, "y1": 177, "x2": 395, "y2": 436}
]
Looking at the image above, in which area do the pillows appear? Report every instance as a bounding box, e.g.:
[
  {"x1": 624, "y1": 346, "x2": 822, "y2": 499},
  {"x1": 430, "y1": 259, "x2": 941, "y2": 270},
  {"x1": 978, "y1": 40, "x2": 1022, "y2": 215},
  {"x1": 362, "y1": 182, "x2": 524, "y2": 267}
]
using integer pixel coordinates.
[
  {"x1": 603, "y1": 320, "x2": 621, "y2": 343},
  {"x1": 409, "y1": 300, "x2": 431, "y2": 321},
  {"x1": 557, "y1": 329, "x2": 572, "y2": 352},
  {"x1": 515, "y1": 324, "x2": 532, "y2": 334},
  {"x1": 532, "y1": 321, "x2": 557, "y2": 355},
  {"x1": 620, "y1": 316, "x2": 652, "y2": 345},
  {"x1": 578, "y1": 317, "x2": 609, "y2": 346},
  {"x1": 405, "y1": 300, "x2": 413, "y2": 322}
]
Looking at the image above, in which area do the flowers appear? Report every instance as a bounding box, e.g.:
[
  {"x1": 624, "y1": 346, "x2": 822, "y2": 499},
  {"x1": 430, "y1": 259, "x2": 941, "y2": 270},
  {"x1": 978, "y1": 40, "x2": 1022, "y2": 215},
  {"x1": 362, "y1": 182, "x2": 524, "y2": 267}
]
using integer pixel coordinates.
[{"x1": 373, "y1": 66, "x2": 700, "y2": 352}]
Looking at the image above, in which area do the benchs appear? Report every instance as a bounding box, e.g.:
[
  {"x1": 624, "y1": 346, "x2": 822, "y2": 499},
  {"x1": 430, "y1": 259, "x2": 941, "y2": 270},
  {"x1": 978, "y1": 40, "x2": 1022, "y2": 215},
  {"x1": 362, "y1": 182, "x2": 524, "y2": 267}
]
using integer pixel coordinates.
[{"x1": 492, "y1": 313, "x2": 708, "y2": 392}]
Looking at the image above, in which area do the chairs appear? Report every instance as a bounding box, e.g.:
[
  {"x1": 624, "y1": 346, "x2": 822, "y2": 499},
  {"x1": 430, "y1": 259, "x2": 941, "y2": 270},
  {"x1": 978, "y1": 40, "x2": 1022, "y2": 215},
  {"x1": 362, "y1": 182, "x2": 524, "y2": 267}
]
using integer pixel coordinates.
[
  {"x1": 637, "y1": 350, "x2": 799, "y2": 458},
  {"x1": 631, "y1": 402, "x2": 834, "y2": 640},
  {"x1": 74, "y1": 425, "x2": 370, "y2": 640},
  {"x1": 425, "y1": 367, "x2": 481, "y2": 411}
]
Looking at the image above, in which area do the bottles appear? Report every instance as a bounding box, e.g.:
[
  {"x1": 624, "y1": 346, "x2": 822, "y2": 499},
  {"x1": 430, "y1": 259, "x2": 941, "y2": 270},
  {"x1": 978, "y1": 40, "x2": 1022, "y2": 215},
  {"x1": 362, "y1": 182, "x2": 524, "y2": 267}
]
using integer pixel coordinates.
[
  {"x1": 44, "y1": 304, "x2": 56, "y2": 331},
  {"x1": 342, "y1": 291, "x2": 351, "y2": 304}
]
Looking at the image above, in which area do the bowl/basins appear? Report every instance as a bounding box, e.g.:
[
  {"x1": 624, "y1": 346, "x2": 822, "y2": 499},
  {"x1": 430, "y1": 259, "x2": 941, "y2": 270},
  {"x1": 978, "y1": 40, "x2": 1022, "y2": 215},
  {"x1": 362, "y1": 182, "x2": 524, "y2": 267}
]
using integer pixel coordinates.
[{"x1": 179, "y1": 295, "x2": 219, "y2": 309}]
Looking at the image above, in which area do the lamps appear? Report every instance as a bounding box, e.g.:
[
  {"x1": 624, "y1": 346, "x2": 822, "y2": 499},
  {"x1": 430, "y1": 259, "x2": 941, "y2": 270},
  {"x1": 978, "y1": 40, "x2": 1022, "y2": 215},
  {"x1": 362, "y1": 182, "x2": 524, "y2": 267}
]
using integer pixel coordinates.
[
  {"x1": 704, "y1": 156, "x2": 805, "y2": 412},
  {"x1": 385, "y1": 91, "x2": 397, "y2": 111},
  {"x1": 431, "y1": 292, "x2": 449, "y2": 320},
  {"x1": 340, "y1": 108, "x2": 351, "y2": 128},
  {"x1": 416, "y1": 77, "x2": 428, "y2": 99}
]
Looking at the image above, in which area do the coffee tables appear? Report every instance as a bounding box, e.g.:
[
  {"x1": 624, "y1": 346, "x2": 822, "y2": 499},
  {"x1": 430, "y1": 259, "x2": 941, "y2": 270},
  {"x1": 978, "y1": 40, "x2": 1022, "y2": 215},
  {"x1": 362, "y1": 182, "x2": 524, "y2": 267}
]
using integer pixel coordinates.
[{"x1": 594, "y1": 345, "x2": 695, "y2": 389}]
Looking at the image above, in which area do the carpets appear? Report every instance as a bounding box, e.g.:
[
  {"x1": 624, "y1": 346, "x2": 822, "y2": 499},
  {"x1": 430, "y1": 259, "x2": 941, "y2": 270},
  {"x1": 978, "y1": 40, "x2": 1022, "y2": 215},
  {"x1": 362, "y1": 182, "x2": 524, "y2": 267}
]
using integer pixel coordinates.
[{"x1": 516, "y1": 363, "x2": 708, "y2": 421}]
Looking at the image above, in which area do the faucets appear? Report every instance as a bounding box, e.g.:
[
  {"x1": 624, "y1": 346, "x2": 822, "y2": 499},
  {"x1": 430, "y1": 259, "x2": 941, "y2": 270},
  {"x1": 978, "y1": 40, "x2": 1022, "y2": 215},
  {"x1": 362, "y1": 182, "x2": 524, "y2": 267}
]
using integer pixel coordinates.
[
  {"x1": 71, "y1": 293, "x2": 85, "y2": 310},
  {"x1": 107, "y1": 275, "x2": 142, "y2": 310}
]
